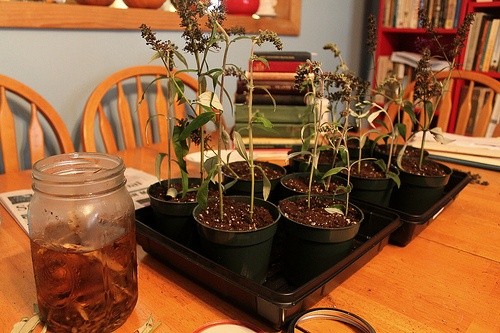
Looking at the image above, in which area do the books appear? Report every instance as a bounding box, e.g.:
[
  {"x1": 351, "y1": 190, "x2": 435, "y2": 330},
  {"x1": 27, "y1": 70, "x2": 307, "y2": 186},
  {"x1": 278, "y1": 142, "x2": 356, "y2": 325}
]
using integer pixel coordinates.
[
  {"x1": 463, "y1": 11, "x2": 500, "y2": 73},
  {"x1": 375, "y1": 51, "x2": 454, "y2": 106},
  {"x1": 454, "y1": 86, "x2": 499, "y2": 137},
  {"x1": 234, "y1": 51, "x2": 328, "y2": 148},
  {"x1": 382, "y1": 0, "x2": 463, "y2": 30}
]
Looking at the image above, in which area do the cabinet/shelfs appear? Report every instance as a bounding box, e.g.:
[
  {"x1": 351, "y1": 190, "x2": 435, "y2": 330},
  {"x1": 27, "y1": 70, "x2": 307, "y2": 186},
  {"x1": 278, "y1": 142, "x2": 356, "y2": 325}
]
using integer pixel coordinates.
[{"x1": 368, "y1": 0, "x2": 500, "y2": 137}]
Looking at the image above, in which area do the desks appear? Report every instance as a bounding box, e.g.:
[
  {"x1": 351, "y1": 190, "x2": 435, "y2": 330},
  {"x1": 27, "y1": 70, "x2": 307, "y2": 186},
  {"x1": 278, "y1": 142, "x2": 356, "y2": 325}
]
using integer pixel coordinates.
[{"x1": 0, "y1": 129, "x2": 500, "y2": 333}]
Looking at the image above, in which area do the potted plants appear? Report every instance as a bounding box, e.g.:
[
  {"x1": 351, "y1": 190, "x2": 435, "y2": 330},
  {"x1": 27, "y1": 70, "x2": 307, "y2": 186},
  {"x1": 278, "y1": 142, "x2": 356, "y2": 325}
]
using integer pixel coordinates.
[{"x1": 134, "y1": 0, "x2": 477, "y2": 286}]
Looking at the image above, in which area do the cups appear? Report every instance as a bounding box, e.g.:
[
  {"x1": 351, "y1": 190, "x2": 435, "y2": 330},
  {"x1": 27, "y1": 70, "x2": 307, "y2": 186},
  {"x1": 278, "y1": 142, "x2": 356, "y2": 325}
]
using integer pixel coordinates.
[{"x1": 183, "y1": 149, "x2": 258, "y2": 178}]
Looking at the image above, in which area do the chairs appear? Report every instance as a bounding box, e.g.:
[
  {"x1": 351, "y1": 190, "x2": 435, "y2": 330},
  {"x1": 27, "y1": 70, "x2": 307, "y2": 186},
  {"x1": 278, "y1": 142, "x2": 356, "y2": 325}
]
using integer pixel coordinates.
[
  {"x1": 380, "y1": 70, "x2": 500, "y2": 138},
  {"x1": 0, "y1": 65, "x2": 226, "y2": 175}
]
